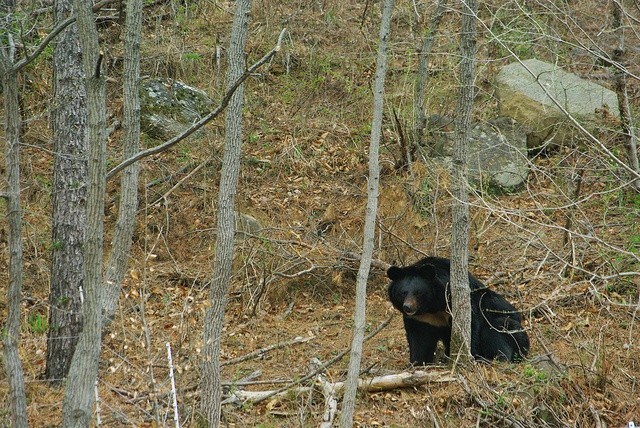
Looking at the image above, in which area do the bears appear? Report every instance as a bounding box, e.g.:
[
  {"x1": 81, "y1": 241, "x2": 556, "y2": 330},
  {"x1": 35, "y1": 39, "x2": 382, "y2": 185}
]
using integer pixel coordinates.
[{"x1": 386, "y1": 255, "x2": 531, "y2": 367}]
